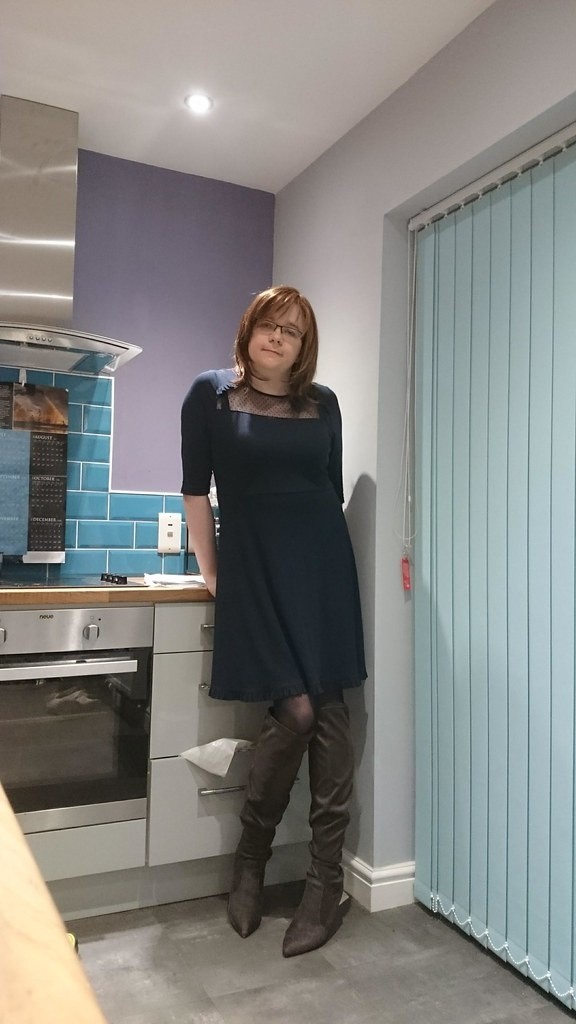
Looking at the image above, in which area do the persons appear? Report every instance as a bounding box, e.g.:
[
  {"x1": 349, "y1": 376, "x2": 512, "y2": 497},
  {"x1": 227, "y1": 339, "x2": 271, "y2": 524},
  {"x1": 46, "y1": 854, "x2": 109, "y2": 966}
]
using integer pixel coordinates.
[{"x1": 181, "y1": 285, "x2": 368, "y2": 957}]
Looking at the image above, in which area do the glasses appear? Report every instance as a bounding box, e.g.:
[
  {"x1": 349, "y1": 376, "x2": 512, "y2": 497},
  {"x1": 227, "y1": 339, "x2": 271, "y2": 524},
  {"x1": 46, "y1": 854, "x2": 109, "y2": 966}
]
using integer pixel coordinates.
[{"x1": 255, "y1": 319, "x2": 307, "y2": 341}]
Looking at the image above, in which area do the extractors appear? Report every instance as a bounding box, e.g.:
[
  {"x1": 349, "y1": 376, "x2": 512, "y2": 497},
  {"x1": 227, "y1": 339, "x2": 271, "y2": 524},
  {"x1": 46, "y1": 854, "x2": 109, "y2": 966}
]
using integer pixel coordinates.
[{"x1": 0, "y1": 318, "x2": 143, "y2": 376}]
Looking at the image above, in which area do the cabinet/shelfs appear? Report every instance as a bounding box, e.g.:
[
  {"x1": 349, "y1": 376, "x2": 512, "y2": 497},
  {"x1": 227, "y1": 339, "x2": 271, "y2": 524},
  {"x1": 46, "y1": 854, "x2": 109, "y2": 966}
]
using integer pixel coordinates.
[{"x1": 147, "y1": 600, "x2": 312, "y2": 868}]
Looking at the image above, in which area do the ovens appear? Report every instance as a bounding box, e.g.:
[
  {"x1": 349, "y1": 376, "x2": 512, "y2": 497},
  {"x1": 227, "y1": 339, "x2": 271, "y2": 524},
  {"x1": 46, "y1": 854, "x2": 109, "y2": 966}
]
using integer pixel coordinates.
[{"x1": 0, "y1": 605, "x2": 155, "y2": 886}]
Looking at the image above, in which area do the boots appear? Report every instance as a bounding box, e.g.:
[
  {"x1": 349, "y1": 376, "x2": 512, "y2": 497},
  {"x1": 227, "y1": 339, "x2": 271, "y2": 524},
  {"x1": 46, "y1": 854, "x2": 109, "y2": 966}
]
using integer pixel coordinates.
[
  {"x1": 282, "y1": 864, "x2": 345, "y2": 958},
  {"x1": 228, "y1": 836, "x2": 273, "y2": 939}
]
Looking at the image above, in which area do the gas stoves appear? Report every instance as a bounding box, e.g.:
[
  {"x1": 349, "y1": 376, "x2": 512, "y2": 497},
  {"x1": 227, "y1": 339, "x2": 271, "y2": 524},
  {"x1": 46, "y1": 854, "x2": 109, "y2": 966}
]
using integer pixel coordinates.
[{"x1": 0, "y1": 572, "x2": 149, "y2": 590}]
